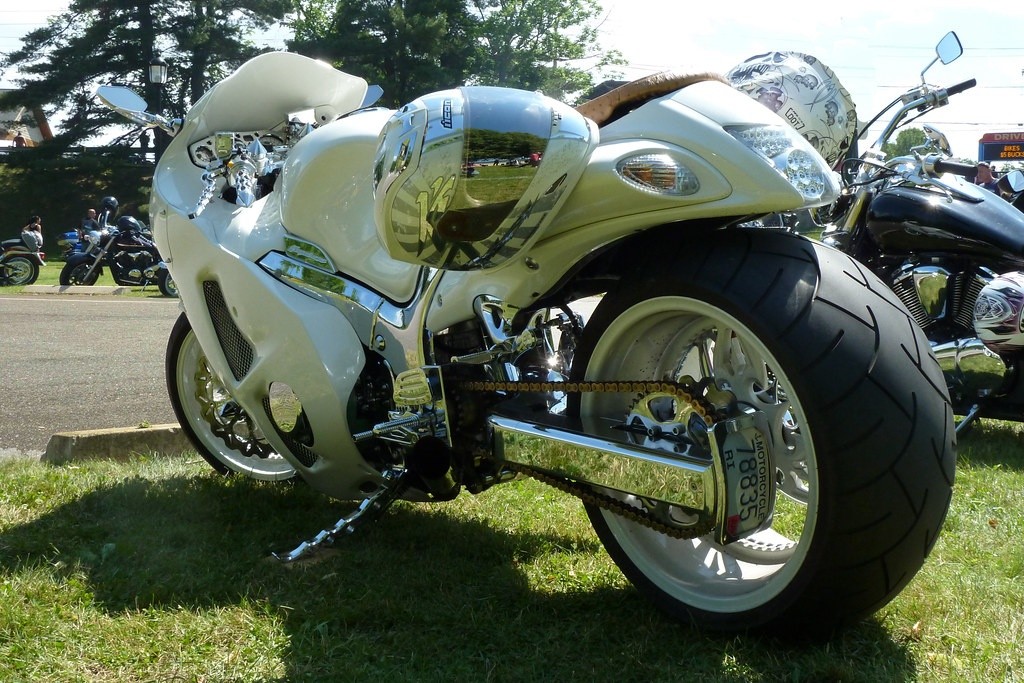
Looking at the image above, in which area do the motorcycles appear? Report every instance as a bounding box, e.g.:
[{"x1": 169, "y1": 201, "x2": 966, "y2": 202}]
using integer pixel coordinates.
[
  {"x1": 0, "y1": 232, "x2": 46, "y2": 288},
  {"x1": 97, "y1": 44, "x2": 956, "y2": 636},
  {"x1": 158, "y1": 268, "x2": 179, "y2": 298},
  {"x1": 59, "y1": 196, "x2": 163, "y2": 290},
  {"x1": 804, "y1": 35, "x2": 1024, "y2": 444}
]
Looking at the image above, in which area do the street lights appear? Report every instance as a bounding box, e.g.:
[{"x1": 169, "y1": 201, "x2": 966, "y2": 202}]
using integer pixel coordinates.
[{"x1": 149, "y1": 49, "x2": 168, "y2": 167}]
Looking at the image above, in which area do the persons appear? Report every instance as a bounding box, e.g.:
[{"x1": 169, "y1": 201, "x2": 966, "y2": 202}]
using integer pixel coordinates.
[
  {"x1": 466, "y1": 151, "x2": 542, "y2": 178},
  {"x1": 23, "y1": 216, "x2": 43, "y2": 250},
  {"x1": 82, "y1": 208, "x2": 102, "y2": 233},
  {"x1": 976, "y1": 162, "x2": 1024, "y2": 212},
  {"x1": 12, "y1": 132, "x2": 28, "y2": 147}
]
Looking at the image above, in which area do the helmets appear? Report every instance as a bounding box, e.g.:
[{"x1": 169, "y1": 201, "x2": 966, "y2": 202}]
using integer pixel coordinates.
[
  {"x1": 375, "y1": 86, "x2": 598, "y2": 275},
  {"x1": 101, "y1": 196, "x2": 120, "y2": 219},
  {"x1": 726, "y1": 51, "x2": 855, "y2": 170}
]
